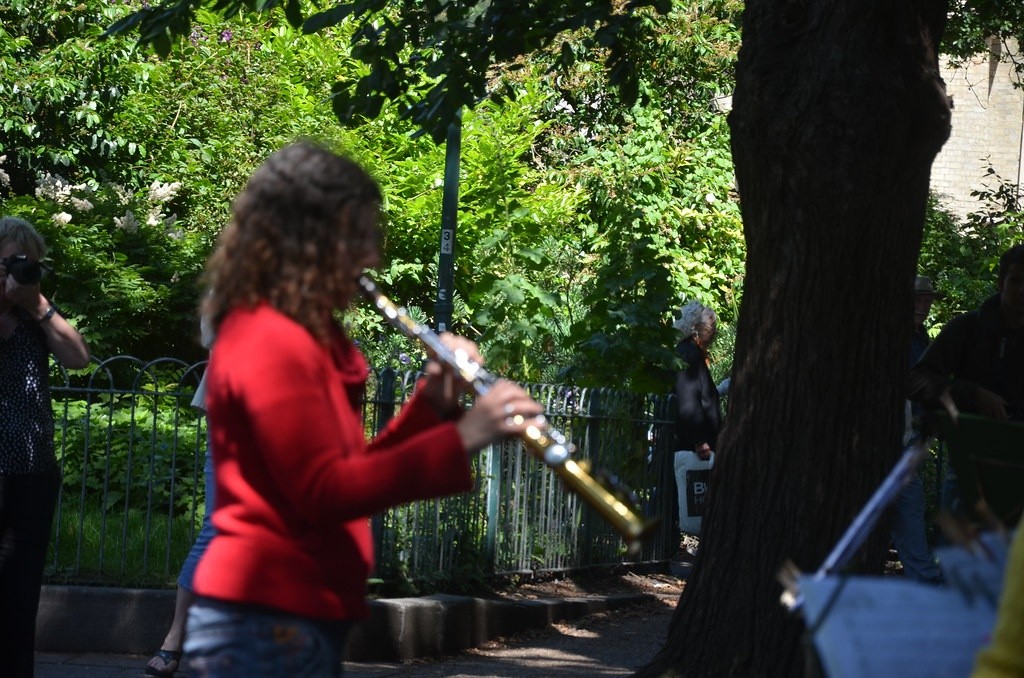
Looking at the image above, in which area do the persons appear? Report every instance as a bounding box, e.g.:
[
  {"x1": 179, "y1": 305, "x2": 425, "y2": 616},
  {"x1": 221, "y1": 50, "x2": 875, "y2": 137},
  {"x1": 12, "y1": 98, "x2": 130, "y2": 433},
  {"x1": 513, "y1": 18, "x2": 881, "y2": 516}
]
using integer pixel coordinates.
[
  {"x1": 0, "y1": 216, "x2": 90, "y2": 678},
  {"x1": 888, "y1": 247, "x2": 1024, "y2": 678},
  {"x1": 665, "y1": 302, "x2": 730, "y2": 559},
  {"x1": 143, "y1": 138, "x2": 545, "y2": 678}
]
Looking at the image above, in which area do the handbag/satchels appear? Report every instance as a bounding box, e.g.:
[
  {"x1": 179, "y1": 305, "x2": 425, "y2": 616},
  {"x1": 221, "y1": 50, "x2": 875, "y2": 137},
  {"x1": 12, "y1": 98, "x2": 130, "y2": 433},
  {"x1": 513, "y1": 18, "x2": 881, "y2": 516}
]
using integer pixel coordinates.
[{"x1": 673, "y1": 448, "x2": 717, "y2": 536}]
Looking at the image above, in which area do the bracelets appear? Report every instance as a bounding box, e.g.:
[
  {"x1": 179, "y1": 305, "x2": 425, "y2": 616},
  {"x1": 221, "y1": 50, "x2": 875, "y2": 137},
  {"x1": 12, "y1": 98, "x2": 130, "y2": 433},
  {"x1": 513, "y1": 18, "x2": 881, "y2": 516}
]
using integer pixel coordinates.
[{"x1": 37, "y1": 307, "x2": 56, "y2": 326}]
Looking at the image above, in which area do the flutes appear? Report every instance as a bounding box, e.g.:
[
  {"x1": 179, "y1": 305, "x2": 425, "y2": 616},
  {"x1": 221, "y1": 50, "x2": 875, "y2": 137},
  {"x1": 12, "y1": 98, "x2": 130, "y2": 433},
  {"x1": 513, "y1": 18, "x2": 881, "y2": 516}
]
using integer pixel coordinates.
[{"x1": 357, "y1": 273, "x2": 652, "y2": 553}]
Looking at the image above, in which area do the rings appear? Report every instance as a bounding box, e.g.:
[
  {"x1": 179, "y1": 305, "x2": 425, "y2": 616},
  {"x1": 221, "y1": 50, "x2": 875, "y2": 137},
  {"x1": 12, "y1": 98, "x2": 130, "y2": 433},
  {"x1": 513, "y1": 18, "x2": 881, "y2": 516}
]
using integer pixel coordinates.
[{"x1": 504, "y1": 404, "x2": 512, "y2": 419}]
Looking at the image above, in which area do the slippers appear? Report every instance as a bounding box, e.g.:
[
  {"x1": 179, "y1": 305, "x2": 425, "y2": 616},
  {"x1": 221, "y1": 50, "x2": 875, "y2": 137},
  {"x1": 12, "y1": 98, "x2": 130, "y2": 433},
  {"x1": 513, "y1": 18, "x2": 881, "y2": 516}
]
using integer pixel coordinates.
[{"x1": 145, "y1": 649, "x2": 181, "y2": 677}]
